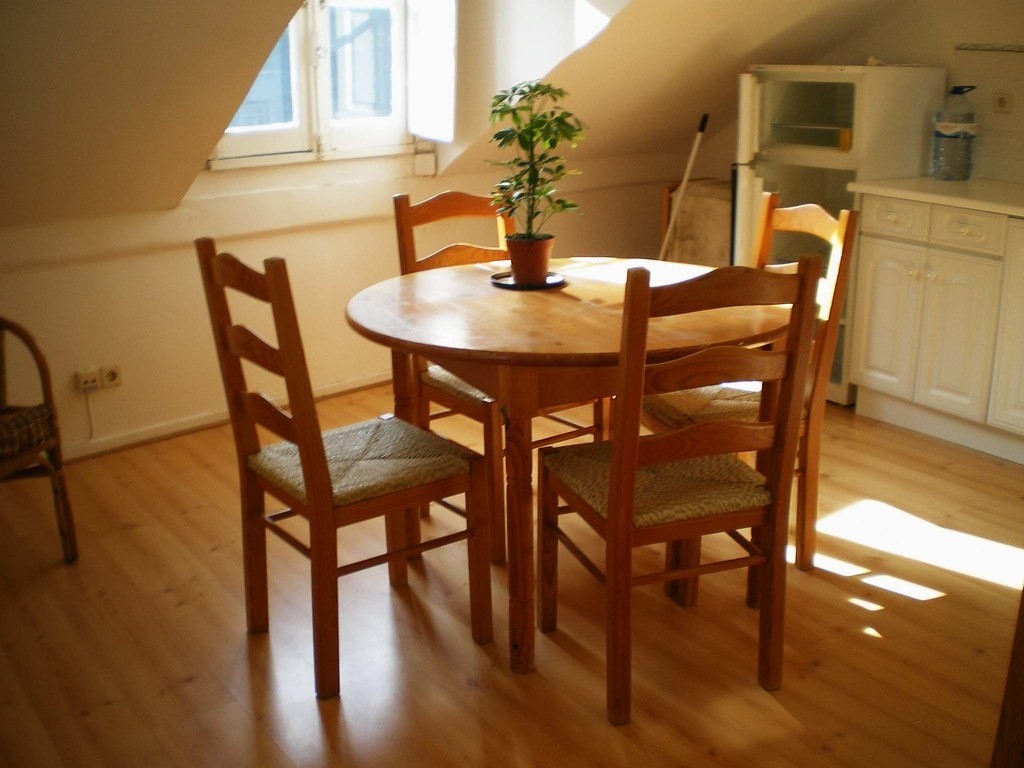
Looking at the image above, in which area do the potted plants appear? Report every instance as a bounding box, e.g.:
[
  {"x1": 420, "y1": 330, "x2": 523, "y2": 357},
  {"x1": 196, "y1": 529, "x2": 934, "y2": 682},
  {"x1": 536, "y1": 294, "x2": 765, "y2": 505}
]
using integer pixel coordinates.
[{"x1": 487, "y1": 75, "x2": 589, "y2": 290}]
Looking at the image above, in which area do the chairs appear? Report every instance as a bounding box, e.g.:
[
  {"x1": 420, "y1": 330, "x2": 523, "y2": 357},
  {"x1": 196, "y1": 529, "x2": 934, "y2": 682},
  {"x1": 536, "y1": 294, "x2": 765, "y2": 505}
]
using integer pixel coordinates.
[
  {"x1": 394, "y1": 187, "x2": 615, "y2": 562},
  {"x1": 642, "y1": 191, "x2": 860, "y2": 594},
  {"x1": 535, "y1": 253, "x2": 821, "y2": 725},
  {"x1": 193, "y1": 236, "x2": 493, "y2": 701},
  {"x1": 0, "y1": 315, "x2": 79, "y2": 565}
]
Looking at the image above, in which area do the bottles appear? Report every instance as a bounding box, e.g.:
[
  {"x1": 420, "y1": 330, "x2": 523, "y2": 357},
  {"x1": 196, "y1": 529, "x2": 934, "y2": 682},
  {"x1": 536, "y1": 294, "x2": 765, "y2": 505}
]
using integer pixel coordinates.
[{"x1": 931, "y1": 86, "x2": 978, "y2": 181}]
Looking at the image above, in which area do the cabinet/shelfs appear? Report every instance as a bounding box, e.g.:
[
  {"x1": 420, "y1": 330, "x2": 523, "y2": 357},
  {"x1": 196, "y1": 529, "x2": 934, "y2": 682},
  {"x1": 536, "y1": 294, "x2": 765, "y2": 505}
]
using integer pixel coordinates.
[{"x1": 738, "y1": 64, "x2": 1024, "y2": 463}]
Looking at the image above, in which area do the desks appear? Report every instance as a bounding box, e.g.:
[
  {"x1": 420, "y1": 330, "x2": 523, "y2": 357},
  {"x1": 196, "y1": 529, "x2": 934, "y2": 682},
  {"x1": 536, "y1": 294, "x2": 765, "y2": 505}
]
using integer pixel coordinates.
[{"x1": 354, "y1": 255, "x2": 795, "y2": 674}]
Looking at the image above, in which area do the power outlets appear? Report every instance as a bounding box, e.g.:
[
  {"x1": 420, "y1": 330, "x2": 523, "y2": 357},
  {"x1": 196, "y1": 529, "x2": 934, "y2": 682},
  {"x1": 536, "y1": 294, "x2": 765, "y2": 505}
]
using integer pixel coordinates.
[
  {"x1": 101, "y1": 365, "x2": 123, "y2": 389},
  {"x1": 76, "y1": 369, "x2": 98, "y2": 391}
]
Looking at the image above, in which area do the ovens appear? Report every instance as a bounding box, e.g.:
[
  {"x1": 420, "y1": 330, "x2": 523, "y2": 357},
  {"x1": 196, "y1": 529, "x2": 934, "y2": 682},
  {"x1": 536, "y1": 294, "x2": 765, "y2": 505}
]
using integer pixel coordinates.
[{"x1": 732, "y1": 62, "x2": 947, "y2": 408}]
